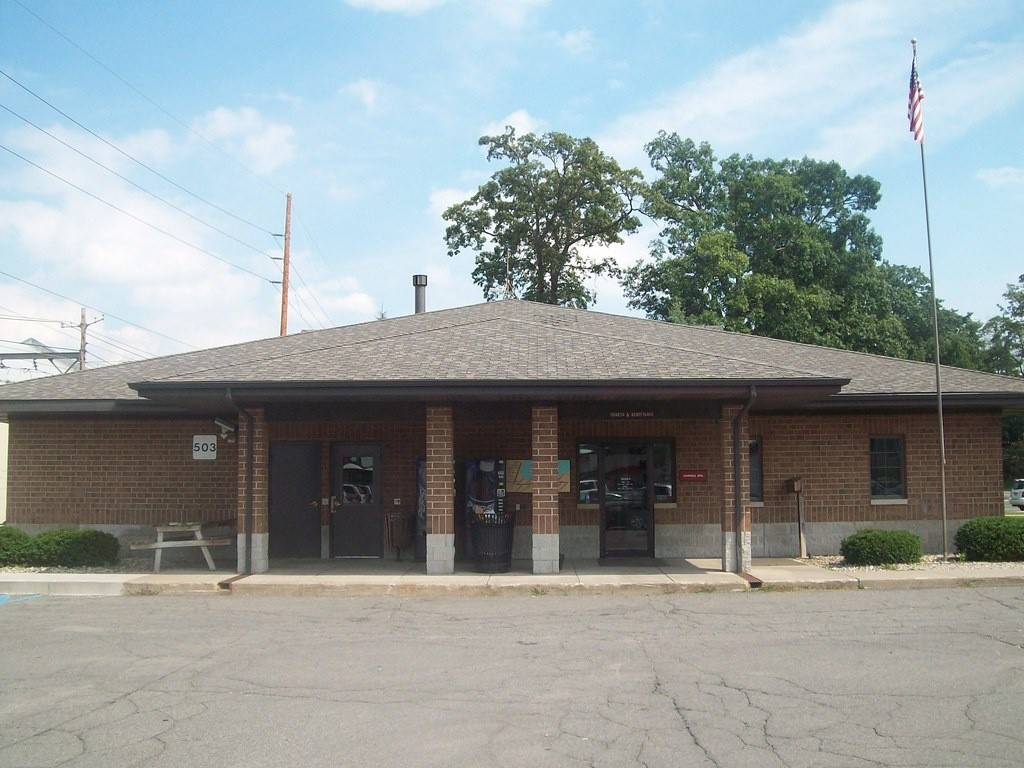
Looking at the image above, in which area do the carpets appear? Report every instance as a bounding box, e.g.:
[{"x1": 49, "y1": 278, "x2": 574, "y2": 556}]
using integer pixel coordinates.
[{"x1": 598, "y1": 557, "x2": 669, "y2": 567}]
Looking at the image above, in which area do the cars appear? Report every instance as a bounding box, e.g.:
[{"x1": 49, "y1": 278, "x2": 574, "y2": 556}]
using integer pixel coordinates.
[
  {"x1": 580, "y1": 478, "x2": 671, "y2": 504},
  {"x1": 343, "y1": 483, "x2": 373, "y2": 504}
]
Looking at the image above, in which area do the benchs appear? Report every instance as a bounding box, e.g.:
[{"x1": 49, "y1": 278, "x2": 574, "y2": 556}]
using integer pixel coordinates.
[{"x1": 130, "y1": 534, "x2": 236, "y2": 550}]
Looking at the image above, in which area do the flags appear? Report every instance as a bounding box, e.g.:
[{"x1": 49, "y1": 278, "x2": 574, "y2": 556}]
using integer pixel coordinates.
[{"x1": 907, "y1": 51, "x2": 926, "y2": 144}]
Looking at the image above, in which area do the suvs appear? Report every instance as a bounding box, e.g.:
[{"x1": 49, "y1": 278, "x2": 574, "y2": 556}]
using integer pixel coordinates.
[{"x1": 1009, "y1": 478, "x2": 1024, "y2": 511}]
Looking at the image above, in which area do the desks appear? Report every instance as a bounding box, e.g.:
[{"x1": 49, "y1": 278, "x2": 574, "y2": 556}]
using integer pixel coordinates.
[{"x1": 151, "y1": 517, "x2": 238, "y2": 573}]
